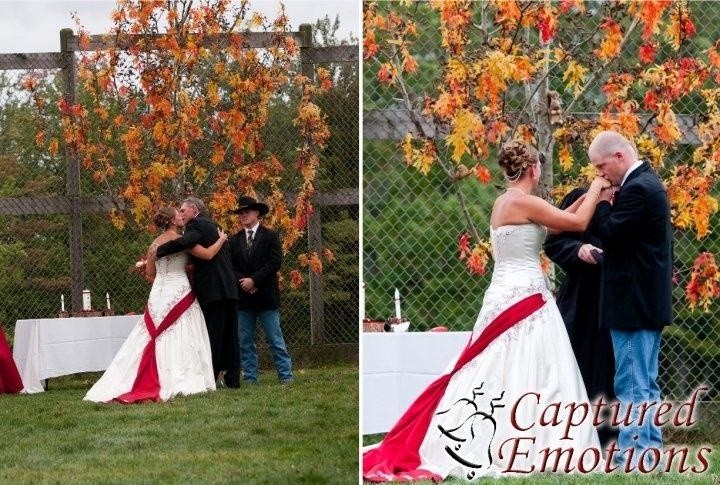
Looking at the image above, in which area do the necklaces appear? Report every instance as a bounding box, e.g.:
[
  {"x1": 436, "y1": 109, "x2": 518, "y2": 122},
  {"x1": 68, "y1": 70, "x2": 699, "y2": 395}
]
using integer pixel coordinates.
[{"x1": 506, "y1": 187, "x2": 525, "y2": 193}]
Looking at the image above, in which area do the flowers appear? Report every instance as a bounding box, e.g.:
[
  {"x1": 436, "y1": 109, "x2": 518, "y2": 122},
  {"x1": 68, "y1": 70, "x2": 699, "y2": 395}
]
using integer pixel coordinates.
[{"x1": 136, "y1": 257, "x2": 147, "y2": 277}]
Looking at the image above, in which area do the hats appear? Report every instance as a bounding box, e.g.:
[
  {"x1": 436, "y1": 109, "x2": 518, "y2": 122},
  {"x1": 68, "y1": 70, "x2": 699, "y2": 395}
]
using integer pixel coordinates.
[{"x1": 228, "y1": 196, "x2": 269, "y2": 216}]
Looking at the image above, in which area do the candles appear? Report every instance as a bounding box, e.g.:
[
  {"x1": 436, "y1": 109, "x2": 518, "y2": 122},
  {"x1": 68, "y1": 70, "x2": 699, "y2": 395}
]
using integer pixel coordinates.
[
  {"x1": 61, "y1": 294, "x2": 65, "y2": 311},
  {"x1": 394, "y1": 288, "x2": 401, "y2": 319},
  {"x1": 106, "y1": 293, "x2": 111, "y2": 309}
]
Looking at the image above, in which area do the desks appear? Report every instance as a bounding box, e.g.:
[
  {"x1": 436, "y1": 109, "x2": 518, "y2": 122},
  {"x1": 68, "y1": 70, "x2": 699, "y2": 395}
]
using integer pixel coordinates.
[
  {"x1": 363, "y1": 331, "x2": 473, "y2": 435},
  {"x1": 13, "y1": 315, "x2": 143, "y2": 395}
]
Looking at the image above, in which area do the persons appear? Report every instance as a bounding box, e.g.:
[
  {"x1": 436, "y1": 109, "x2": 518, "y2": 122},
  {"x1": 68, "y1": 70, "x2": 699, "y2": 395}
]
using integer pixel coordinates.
[
  {"x1": 588, "y1": 130, "x2": 674, "y2": 465},
  {"x1": 487, "y1": 137, "x2": 611, "y2": 478},
  {"x1": 142, "y1": 206, "x2": 229, "y2": 398},
  {"x1": 541, "y1": 185, "x2": 618, "y2": 446},
  {"x1": 148, "y1": 198, "x2": 242, "y2": 387},
  {"x1": 229, "y1": 195, "x2": 293, "y2": 386}
]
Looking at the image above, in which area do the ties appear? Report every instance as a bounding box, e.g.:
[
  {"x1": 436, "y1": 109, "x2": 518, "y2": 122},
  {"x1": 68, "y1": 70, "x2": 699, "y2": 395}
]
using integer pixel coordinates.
[{"x1": 246, "y1": 230, "x2": 254, "y2": 257}]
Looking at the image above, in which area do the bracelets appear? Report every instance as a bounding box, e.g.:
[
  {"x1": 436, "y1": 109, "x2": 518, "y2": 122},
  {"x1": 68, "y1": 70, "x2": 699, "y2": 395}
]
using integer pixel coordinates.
[
  {"x1": 589, "y1": 189, "x2": 600, "y2": 197},
  {"x1": 218, "y1": 238, "x2": 223, "y2": 244}
]
